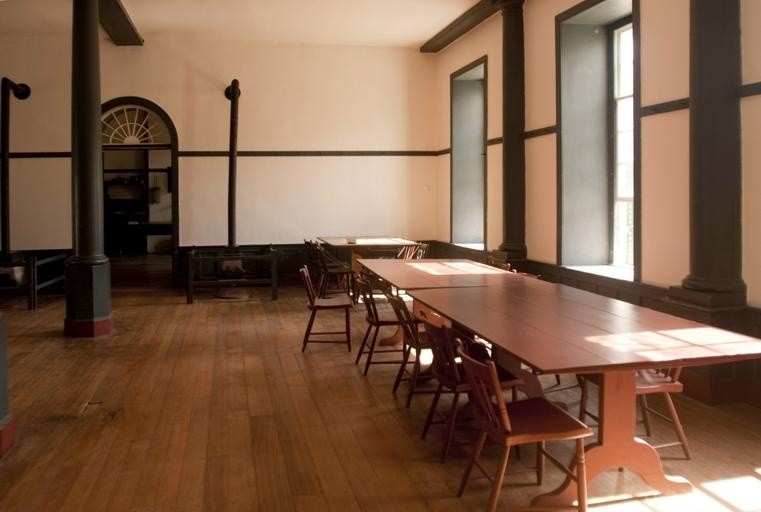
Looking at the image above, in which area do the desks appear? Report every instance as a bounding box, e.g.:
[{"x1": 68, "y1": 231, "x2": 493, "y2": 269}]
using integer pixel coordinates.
[{"x1": 409, "y1": 280, "x2": 761, "y2": 510}]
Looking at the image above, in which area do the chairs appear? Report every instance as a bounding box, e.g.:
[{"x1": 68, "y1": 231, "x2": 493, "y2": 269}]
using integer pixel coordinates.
[{"x1": 291, "y1": 239, "x2": 694, "y2": 510}]
